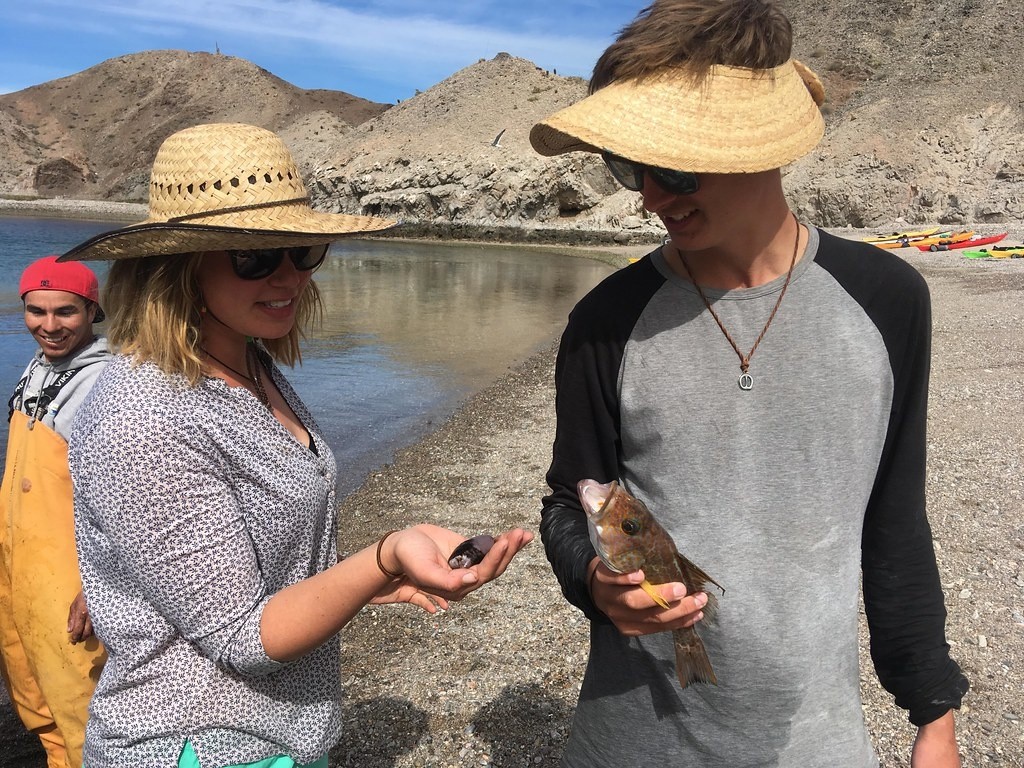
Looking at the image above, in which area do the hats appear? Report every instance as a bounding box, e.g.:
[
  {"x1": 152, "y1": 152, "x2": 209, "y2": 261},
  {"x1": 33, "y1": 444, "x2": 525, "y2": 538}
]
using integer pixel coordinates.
[
  {"x1": 20, "y1": 256, "x2": 105, "y2": 323},
  {"x1": 529, "y1": 56, "x2": 825, "y2": 175},
  {"x1": 54, "y1": 123, "x2": 399, "y2": 263}
]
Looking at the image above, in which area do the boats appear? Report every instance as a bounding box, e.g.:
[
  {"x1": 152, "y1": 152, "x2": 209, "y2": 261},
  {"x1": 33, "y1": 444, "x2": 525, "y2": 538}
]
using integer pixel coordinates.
[
  {"x1": 873, "y1": 229, "x2": 976, "y2": 249},
  {"x1": 987, "y1": 245, "x2": 1024, "y2": 259},
  {"x1": 963, "y1": 249, "x2": 990, "y2": 258},
  {"x1": 860, "y1": 226, "x2": 940, "y2": 242},
  {"x1": 916, "y1": 231, "x2": 1010, "y2": 252}
]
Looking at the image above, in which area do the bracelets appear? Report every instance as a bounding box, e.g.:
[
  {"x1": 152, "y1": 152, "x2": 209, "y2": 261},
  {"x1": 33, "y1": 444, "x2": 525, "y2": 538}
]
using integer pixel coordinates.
[{"x1": 377, "y1": 530, "x2": 405, "y2": 579}]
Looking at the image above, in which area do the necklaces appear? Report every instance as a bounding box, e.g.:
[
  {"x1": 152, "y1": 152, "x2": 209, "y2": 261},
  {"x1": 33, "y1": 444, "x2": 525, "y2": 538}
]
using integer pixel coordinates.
[
  {"x1": 677, "y1": 210, "x2": 800, "y2": 389},
  {"x1": 197, "y1": 336, "x2": 276, "y2": 419}
]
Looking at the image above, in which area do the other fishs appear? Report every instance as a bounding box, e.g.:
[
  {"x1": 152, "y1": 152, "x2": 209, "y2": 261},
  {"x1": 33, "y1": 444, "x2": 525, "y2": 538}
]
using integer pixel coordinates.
[{"x1": 577, "y1": 477, "x2": 725, "y2": 691}]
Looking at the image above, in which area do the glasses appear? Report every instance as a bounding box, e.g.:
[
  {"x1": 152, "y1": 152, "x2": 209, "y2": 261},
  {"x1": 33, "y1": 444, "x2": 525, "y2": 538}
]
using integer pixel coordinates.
[
  {"x1": 602, "y1": 151, "x2": 699, "y2": 194},
  {"x1": 224, "y1": 243, "x2": 330, "y2": 280}
]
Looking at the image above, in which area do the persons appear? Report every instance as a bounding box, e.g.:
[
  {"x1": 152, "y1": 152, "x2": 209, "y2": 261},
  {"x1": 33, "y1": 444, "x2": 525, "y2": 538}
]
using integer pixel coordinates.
[
  {"x1": 0, "y1": 255, "x2": 108, "y2": 768},
  {"x1": 527, "y1": 0, "x2": 971, "y2": 768},
  {"x1": 55, "y1": 122, "x2": 536, "y2": 768}
]
